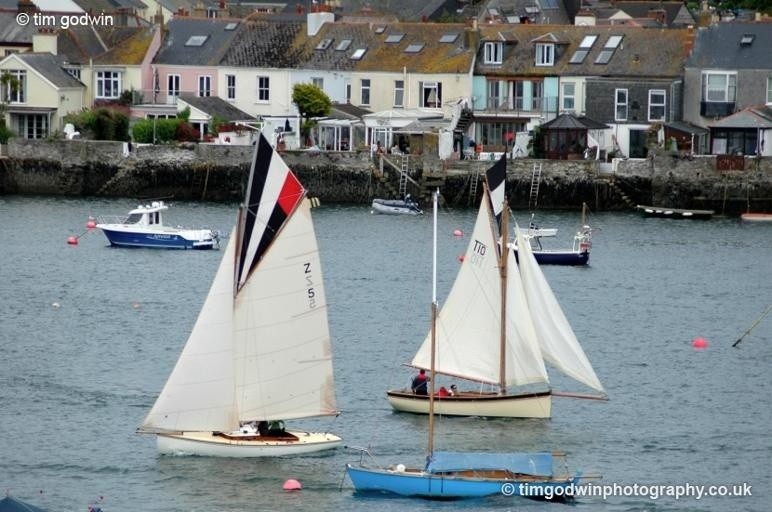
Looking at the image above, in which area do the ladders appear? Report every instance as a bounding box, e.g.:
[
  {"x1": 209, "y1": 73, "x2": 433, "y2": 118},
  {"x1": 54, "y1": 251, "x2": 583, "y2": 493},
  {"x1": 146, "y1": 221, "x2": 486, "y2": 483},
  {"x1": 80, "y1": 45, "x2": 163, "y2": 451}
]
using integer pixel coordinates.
[
  {"x1": 529, "y1": 161, "x2": 543, "y2": 209},
  {"x1": 467, "y1": 161, "x2": 480, "y2": 208},
  {"x1": 399, "y1": 153, "x2": 408, "y2": 200}
]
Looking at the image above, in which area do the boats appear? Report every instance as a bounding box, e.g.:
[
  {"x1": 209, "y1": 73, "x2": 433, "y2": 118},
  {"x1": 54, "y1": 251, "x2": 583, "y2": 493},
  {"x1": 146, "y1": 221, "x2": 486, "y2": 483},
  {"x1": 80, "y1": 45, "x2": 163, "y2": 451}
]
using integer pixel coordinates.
[
  {"x1": 346, "y1": 186, "x2": 611, "y2": 498},
  {"x1": 497, "y1": 202, "x2": 592, "y2": 266},
  {"x1": 94, "y1": 200, "x2": 221, "y2": 251},
  {"x1": 637, "y1": 204, "x2": 772, "y2": 223},
  {"x1": 373, "y1": 197, "x2": 422, "y2": 217}
]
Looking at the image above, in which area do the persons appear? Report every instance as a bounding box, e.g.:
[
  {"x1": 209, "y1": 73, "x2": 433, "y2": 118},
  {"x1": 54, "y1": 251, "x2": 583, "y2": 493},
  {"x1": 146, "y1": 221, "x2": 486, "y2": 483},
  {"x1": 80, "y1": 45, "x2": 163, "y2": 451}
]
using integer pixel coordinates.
[
  {"x1": 255, "y1": 420, "x2": 270, "y2": 436},
  {"x1": 449, "y1": 385, "x2": 459, "y2": 396},
  {"x1": 399, "y1": 135, "x2": 409, "y2": 154},
  {"x1": 470, "y1": 139, "x2": 477, "y2": 153},
  {"x1": 411, "y1": 369, "x2": 431, "y2": 396},
  {"x1": 63, "y1": 121, "x2": 75, "y2": 140},
  {"x1": 669, "y1": 136, "x2": 678, "y2": 151},
  {"x1": 268, "y1": 420, "x2": 286, "y2": 434}
]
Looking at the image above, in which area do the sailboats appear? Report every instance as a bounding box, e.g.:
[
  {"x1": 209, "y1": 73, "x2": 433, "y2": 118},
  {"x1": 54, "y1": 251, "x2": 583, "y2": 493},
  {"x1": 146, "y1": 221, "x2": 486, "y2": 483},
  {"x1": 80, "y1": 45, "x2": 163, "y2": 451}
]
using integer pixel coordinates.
[
  {"x1": 135, "y1": 130, "x2": 342, "y2": 459},
  {"x1": 387, "y1": 146, "x2": 609, "y2": 420}
]
